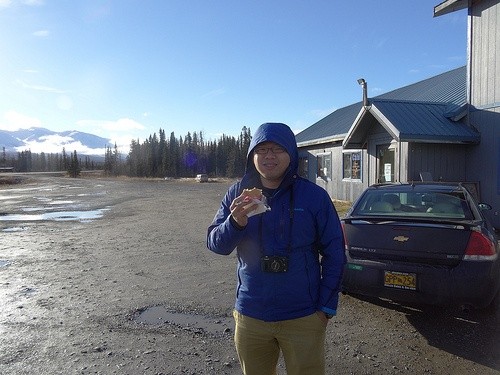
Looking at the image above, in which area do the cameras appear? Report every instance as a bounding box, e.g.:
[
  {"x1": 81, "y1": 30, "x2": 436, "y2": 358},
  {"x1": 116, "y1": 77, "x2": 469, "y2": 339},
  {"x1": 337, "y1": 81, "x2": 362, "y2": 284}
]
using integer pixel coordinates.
[{"x1": 260, "y1": 255, "x2": 288, "y2": 272}]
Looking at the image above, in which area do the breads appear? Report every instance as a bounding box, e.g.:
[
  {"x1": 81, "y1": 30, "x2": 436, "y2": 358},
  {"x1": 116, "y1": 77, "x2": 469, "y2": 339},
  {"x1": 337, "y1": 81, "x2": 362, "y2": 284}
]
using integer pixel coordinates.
[{"x1": 239, "y1": 187, "x2": 262, "y2": 202}]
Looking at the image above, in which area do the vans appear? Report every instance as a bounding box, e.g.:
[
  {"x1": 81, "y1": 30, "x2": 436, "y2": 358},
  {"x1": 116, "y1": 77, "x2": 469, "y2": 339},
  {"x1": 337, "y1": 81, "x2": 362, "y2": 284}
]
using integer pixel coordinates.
[{"x1": 196, "y1": 173, "x2": 208, "y2": 183}]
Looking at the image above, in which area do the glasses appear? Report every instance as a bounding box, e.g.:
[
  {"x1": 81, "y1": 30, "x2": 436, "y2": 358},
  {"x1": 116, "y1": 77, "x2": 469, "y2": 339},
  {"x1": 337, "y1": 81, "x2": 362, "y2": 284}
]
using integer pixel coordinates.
[{"x1": 253, "y1": 147, "x2": 287, "y2": 154}]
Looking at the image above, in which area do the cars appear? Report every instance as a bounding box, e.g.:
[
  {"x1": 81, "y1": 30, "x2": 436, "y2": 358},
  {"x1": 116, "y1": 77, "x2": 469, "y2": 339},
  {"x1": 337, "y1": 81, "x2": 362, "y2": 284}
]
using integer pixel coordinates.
[{"x1": 340, "y1": 181, "x2": 500, "y2": 314}]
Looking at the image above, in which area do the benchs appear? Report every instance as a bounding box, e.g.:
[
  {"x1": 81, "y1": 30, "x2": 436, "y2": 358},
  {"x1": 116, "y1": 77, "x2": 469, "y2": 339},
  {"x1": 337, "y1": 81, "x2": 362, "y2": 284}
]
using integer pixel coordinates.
[{"x1": 374, "y1": 201, "x2": 458, "y2": 214}]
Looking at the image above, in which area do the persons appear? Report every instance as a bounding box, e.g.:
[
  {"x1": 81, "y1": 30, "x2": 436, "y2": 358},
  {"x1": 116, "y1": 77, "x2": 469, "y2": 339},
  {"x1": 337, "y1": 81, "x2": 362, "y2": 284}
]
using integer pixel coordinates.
[{"x1": 206, "y1": 122, "x2": 348, "y2": 375}]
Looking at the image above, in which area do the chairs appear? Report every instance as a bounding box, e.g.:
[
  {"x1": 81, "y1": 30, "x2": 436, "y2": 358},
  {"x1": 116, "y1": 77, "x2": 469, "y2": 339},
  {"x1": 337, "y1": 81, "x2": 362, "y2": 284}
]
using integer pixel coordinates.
[
  {"x1": 384, "y1": 194, "x2": 401, "y2": 208},
  {"x1": 436, "y1": 195, "x2": 453, "y2": 204}
]
